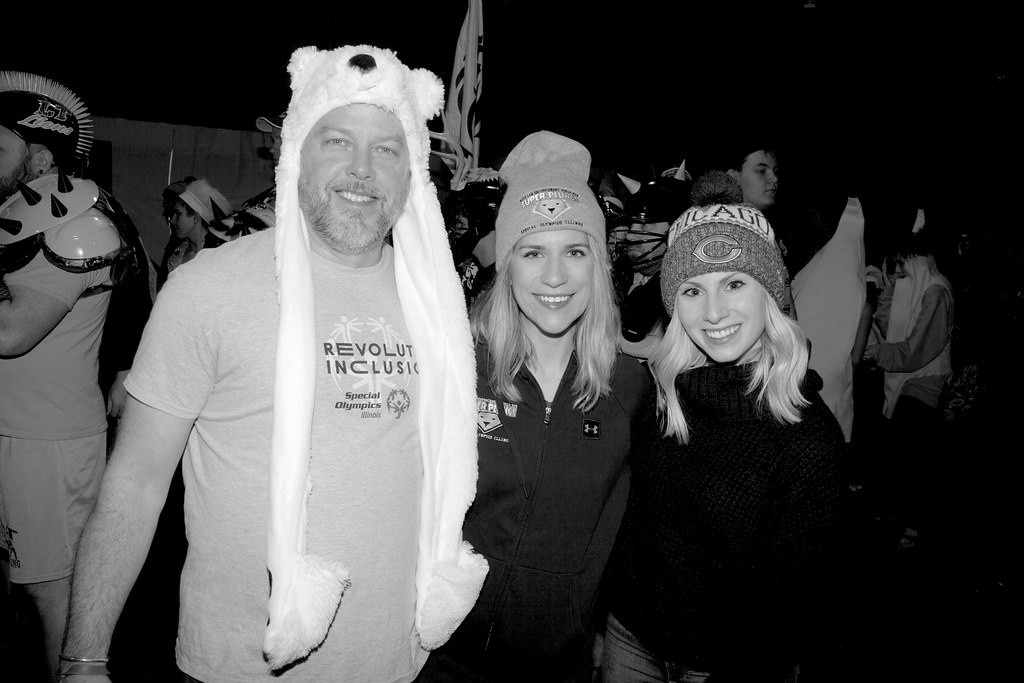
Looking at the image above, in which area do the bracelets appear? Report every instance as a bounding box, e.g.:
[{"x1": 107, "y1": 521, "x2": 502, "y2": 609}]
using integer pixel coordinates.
[{"x1": 60, "y1": 654, "x2": 110, "y2": 676}]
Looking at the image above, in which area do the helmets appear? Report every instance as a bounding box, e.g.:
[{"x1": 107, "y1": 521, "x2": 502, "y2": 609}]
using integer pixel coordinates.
[{"x1": 619, "y1": 160, "x2": 695, "y2": 278}]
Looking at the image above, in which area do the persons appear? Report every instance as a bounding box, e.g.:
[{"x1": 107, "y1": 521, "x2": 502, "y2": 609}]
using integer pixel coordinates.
[
  {"x1": 852, "y1": 246, "x2": 880, "y2": 380},
  {"x1": 723, "y1": 140, "x2": 779, "y2": 211},
  {"x1": 0, "y1": 70, "x2": 122, "y2": 683},
  {"x1": 862, "y1": 230, "x2": 955, "y2": 549},
  {"x1": 155, "y1": 176, "x2": 196, "y2": 294},
  {"x1": 59, "y1": 43, "x2": 488, "y2": 683},
  {"x1": 427, "y1": 131, "x2": 654, "y2": 683},
  {"x1": 582, "y1": 173, "x2": 851, "y2": 680},
  {"x1": 164, "y1": 177, "x2": 234, "y2": 282}
]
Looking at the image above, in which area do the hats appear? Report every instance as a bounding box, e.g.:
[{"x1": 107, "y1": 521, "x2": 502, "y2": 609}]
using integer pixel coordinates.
[
  {"x1": 494, "y1": 130, "x2": 606, "y2": 272},
  {"x1": 277, "y1": 46, "x2": 444, "y2": 184},
  {"x1": 660, "y1": 172, "x2": 786, "y2": 313},
  {"x1": 162, "y1": 175, "x2": 197, "y2": 204},
  {"x1": 0, "y1": 70, "x2": 94, "y2": 172},
  {"x1": 178, "y1": 179, "x2": 234, "y2": 225},
  {"x1": 256, "y1": 110, "x2": 287, "y2": 133}
]
